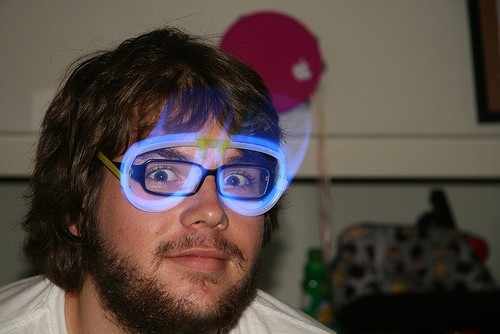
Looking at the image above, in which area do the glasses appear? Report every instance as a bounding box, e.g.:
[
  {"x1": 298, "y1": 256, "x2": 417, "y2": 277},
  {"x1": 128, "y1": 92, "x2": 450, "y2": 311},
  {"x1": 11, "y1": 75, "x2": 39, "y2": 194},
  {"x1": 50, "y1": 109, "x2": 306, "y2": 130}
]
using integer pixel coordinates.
[
  {"x1": 94, "y1": 150, "x2": 275, "y2": 202},
  {"x1": 120, "y1": 131, "x2": 289, "y2": 217}
]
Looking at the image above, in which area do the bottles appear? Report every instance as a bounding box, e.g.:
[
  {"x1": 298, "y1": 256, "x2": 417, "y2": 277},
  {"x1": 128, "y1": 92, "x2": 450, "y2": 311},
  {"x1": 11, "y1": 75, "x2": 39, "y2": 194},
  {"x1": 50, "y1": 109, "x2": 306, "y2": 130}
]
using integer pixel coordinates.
[{"x1": 304, "y1": 247, "x2": 340, "y2": 333}]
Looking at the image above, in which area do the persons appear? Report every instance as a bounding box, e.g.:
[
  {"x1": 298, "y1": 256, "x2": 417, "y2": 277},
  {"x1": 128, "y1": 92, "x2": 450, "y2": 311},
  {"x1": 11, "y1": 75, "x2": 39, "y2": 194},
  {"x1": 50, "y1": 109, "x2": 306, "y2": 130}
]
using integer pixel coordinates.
[{"x1": 0, "y1": 25, "x2": 341, "y2": 334}]
